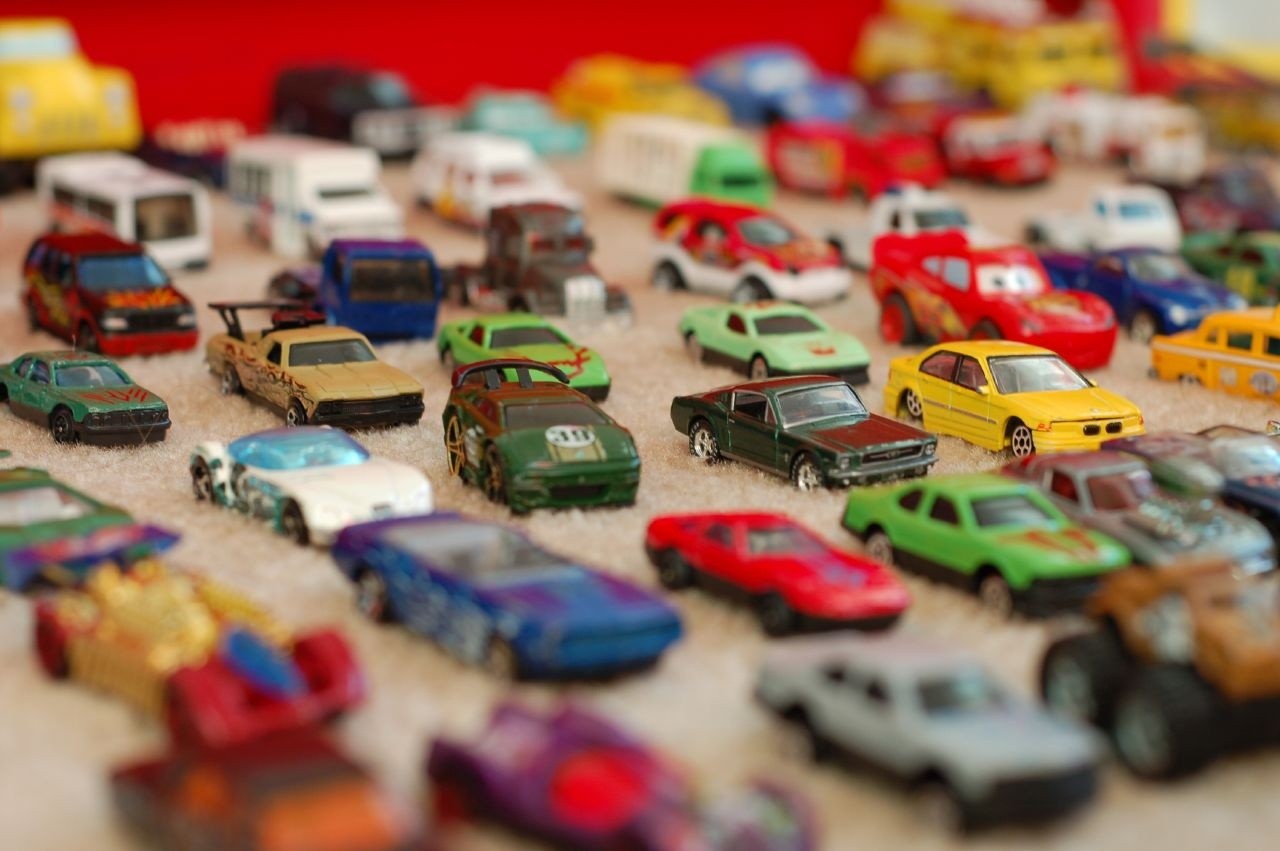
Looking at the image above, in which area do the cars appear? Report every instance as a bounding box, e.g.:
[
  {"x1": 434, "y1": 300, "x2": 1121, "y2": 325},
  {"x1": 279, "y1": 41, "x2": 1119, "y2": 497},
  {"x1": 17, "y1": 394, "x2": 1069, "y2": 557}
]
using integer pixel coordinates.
[
  {"x1": 756, "y1": 630, "x2": 1111, "y2": 842},
  {"x1": 330, "y1": 507, "x2": 682, "y2": 694},
  {"x1": 105, "y1": 731, "x2": 417, "y2": 851},
  {"x1": 667, "y1": 374, "x2": 941, "y2": 488},
  {"x1": 434, "y1": 315, "x2": 612, "y2": 408},
  {"x1": 1, "y1": 348, "x2": 174, "y2": 450},
  {"x1": 885, "y1": 337, "x2": 1147, "y2": 465},
  {"x1": 676, "y1": 297, "x2": 870, "y2": 390},
  {"x1": 26, "y1": 554, "x2": 371, "y2": 748},
  {"x1": 1145, "y1": 304, "x2": 1280, "y2": 399},
  {"x1": 444, "y1": 357, "x2": 644, "y2": 520},
  {"x1": 1096, "y1": 430, "x2": 1280, "y2": 539},
  {"x1": 0, "y1": 446, "x2": 176, "y2": 592},
  {"x1": 414, "y1": 690, "x2": 826, "y2": 851},
  {"x1": 837, "y1": 473, "x2": 1133, "y2": 617},
  {"x1": 996, "y1": 445, "x2": 1277, "y2": 583},
  {"x1": 637, "y1": 507, "x2": 914, "y2": 631},
  {"x1": 0, "y1": 0, "x2": 1280, "y2": 373}
]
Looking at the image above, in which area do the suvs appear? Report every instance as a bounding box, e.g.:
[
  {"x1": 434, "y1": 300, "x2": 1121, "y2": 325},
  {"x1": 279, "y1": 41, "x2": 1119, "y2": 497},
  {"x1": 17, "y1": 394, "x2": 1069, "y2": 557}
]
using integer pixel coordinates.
[{"x1": 1035, "y1": 552, "x2": 1280, "y2": 785}]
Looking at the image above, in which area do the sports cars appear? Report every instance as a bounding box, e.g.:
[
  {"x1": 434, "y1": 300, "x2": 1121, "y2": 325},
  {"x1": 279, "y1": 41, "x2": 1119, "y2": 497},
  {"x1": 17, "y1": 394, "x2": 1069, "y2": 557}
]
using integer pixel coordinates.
[{"x1": 188, "y1": 418, "x2": 436, "y2": 550}]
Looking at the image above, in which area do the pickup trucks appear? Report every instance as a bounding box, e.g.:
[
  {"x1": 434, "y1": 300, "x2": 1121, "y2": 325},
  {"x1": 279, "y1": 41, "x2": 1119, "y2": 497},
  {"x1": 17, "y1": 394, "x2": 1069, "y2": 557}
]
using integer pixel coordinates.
[{"x1": 205, "y1": 297, "x2": 428, "y2": 438}]
